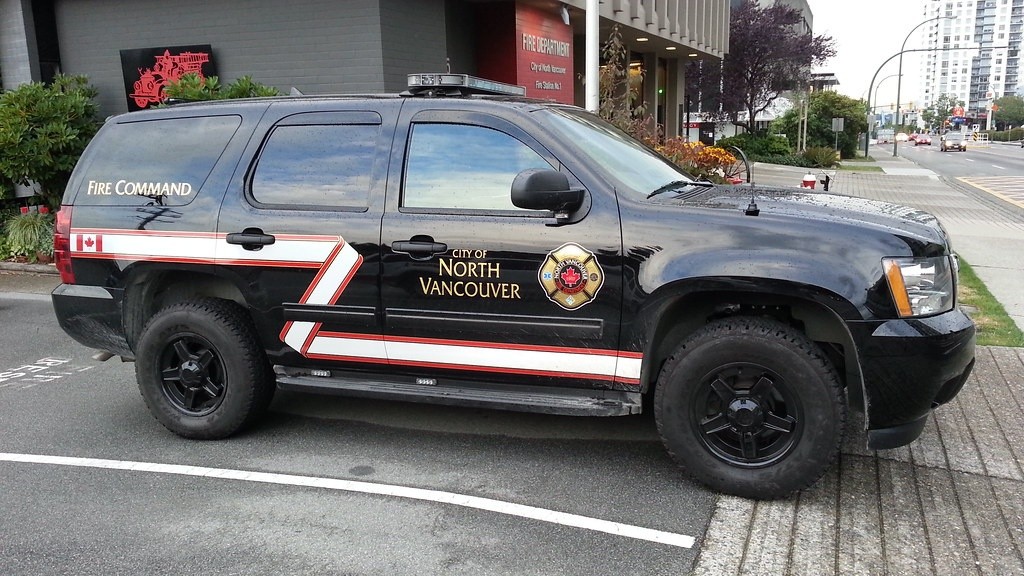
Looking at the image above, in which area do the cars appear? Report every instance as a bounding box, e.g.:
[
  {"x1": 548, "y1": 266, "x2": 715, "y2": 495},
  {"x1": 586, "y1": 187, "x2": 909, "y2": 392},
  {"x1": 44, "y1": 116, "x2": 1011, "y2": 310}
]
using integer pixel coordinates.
[{"x1": 897, "y1": 126, "x2": 969, "y2": 153}]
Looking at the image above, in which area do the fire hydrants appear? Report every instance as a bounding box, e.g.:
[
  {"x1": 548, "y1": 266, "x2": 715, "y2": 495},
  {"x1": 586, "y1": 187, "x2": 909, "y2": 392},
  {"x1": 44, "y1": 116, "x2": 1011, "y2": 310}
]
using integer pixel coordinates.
[{"x1": 802, "y1": 171, "x2": 815, "y2": 186}]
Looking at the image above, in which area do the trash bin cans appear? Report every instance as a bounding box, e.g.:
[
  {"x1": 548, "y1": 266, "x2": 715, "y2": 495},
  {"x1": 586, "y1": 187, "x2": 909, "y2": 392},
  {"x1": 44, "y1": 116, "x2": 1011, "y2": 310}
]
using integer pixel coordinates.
[{"x1": 698, "y1": 121, "x2": 717, "y2": 146}]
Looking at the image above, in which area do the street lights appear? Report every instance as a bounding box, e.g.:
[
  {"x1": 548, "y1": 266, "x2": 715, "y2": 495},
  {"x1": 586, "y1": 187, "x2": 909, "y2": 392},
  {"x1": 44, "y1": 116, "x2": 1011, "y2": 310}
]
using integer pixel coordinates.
[{"x1": 867, "y1": 14, "x2": 960, "y2": 158}]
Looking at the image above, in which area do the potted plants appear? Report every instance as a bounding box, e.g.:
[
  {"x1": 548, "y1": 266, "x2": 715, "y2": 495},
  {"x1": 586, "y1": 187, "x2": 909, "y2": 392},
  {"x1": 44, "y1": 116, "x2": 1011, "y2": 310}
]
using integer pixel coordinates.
[{"x1": 6, "y1": 213, "x2": 55, "y2": 264}]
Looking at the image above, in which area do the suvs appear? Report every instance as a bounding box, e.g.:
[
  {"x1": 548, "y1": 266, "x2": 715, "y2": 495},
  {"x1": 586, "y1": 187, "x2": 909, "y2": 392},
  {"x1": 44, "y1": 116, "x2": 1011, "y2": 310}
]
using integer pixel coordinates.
[{"x1": 53, "y1": 72, "x2": 976, "y2": 502}]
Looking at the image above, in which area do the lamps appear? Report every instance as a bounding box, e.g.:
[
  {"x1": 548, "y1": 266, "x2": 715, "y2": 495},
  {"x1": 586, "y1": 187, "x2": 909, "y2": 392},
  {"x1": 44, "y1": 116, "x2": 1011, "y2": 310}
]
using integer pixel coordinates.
[{"x1": 559, "y1": 3, "x2": 570, "y2": 25}]
